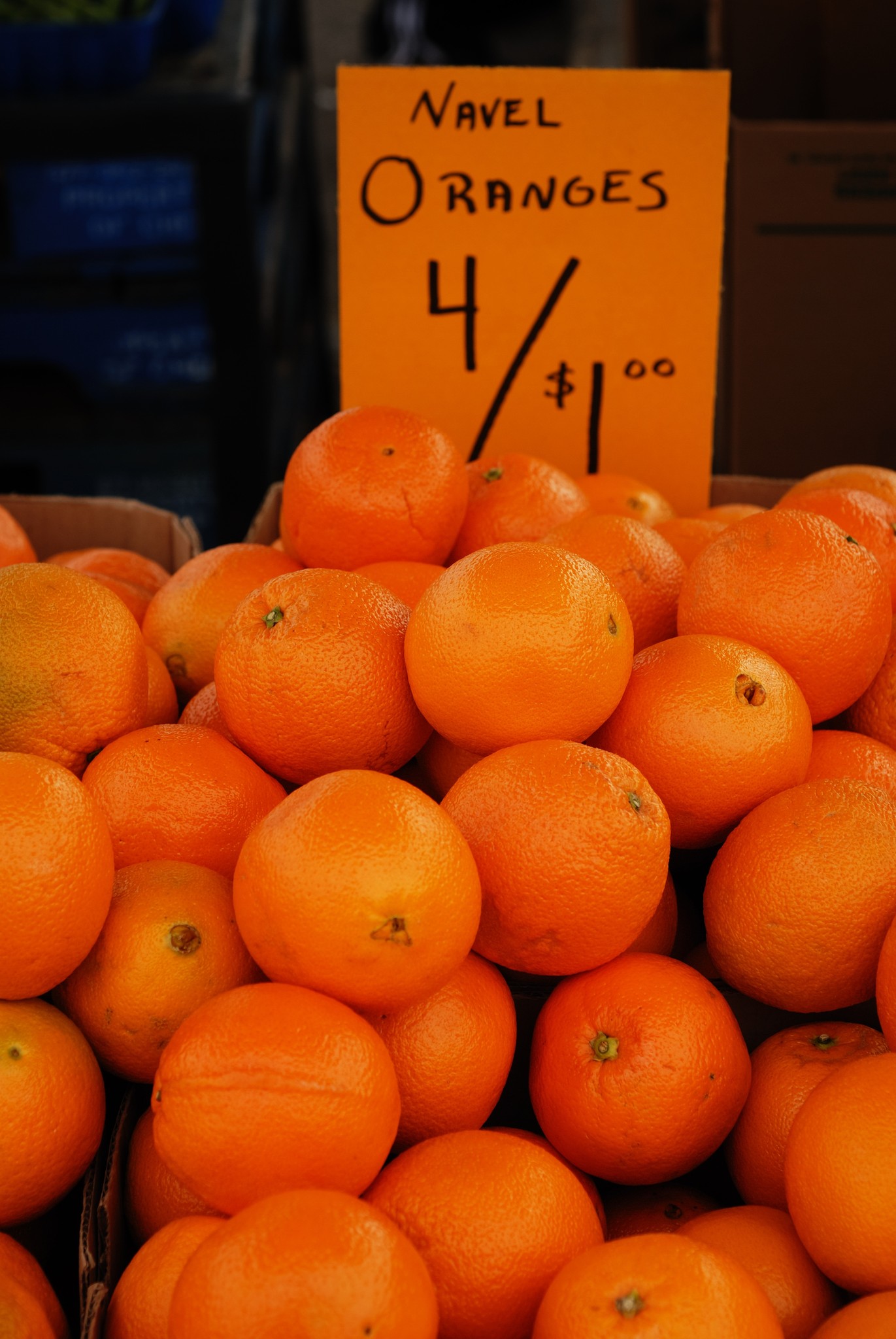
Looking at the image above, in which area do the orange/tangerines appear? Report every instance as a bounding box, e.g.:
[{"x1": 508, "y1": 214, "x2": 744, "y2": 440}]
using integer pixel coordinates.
[{"x1": 0, "y1": 403, "x2": 896, "y2": 1339}]
